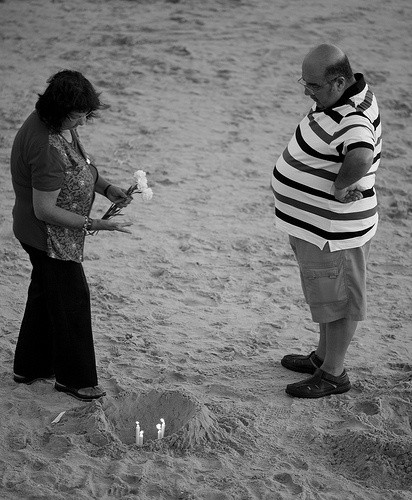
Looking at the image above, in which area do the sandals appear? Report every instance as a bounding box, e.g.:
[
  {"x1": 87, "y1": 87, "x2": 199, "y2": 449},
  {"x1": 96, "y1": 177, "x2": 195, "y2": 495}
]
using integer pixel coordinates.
[
  {"x1": 280, "y1": 351, "x2": 324, "y2": 375},
  {"x1": 286, "y1": 367, "x2": 352, "y2": 398}
]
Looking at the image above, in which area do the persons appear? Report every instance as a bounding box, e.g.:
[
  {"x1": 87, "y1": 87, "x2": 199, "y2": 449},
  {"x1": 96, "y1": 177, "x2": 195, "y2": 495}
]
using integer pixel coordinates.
[
  {"x1": 271, "y1": 42, "x2": 382, "y2": 399},
  {"x1": 9, "y1": 70, "x2": 133, "y2": 402}
]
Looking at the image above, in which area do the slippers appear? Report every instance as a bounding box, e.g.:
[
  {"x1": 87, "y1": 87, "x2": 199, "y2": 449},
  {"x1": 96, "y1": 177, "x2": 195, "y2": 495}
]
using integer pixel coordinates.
[
  {"x1": 13, "y1": 368, "x2": 54, "y2": 385},
  {"x1": 52, "y1": 379, "x2": 106, "y2": 402}
]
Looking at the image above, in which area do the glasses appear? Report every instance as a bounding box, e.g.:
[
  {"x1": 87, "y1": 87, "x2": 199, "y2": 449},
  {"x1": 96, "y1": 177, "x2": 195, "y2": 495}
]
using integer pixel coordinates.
[{"x1": 298, "y1": 76, "x2": 337, "y2": 94}]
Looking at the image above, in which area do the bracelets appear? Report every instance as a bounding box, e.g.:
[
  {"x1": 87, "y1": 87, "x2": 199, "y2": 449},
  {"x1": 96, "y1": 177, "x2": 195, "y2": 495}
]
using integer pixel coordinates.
[
  {"x1": 104, "y1": 184, "x2": 111, "y2": 198},
  {"x1": 81, "y1": 216, "x2": 92, "y2": 234}
]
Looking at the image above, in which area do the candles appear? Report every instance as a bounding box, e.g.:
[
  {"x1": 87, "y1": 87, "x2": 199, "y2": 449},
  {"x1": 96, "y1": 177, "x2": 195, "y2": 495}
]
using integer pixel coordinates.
[
  {"x1": 135, "y1": 421, "x2": 141, "y2": 445},
  {"x1": 155, "y1": 424, "x2": 162, "y2": 441},
  {"x1": 139, "y1": 431, "x2": 144, "y2": 446},
  {"x1": 88, "y1": 169, "x2": 153, "y2": 237},
  {"x1": 161, "y1": 418, "x2": 166, "y2": 435}
]
